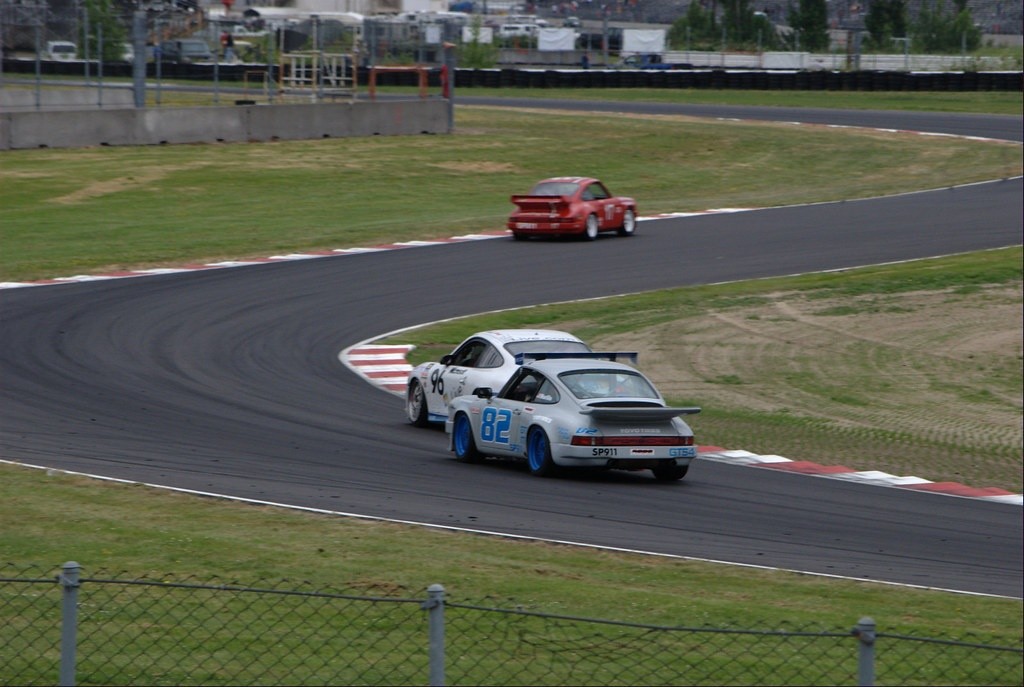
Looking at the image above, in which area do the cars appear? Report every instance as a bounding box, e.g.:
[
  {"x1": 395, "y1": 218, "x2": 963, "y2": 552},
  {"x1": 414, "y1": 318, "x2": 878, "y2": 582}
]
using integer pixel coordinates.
[
  {"x1": 506, "y1": 174, "x2": 642, "y2": 241},
  {"x1": 495, "y1": 13, "x2": 695, "y2": 69},
  {"x1": 447, "y1": 348, "x2": 702, "y2": 485},
  {"x1": 154, "y1": 39, "x2": 220, "y2": 64},
  {"x1": 110, "y1": 42, "x2": 135, "y2": 63},
  {"x1": 46, "y1": 40, "x2": 80, "y2": 61},
  {"x1": 406, "y1": 328, "x2": 598, "y2": 432}
]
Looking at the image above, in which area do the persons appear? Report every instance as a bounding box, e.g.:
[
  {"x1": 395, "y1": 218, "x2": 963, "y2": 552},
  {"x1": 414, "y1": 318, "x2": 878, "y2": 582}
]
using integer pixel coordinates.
[
  {"x1": 221, "y1": 30, "x2": 233, "y2": 62},
  {"x1": 581, "y1": 52, "x2": 589, "y2": 69}
]
